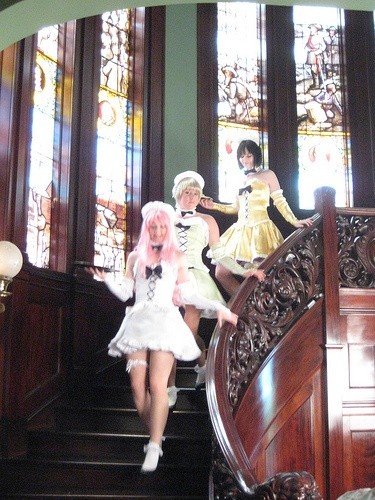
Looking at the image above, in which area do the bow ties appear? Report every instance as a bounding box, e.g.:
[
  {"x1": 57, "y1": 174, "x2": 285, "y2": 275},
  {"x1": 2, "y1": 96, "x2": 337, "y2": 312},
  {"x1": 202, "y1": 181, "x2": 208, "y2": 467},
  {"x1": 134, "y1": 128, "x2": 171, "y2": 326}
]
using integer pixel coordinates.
[
  {"x1": 244, "y1": 169, "x2": 256, "y2": 175},
  {"x1": 176, "y1": 223, "x2": 190, "y2": 230},
  {"x1": 181, "y1": 211, "x2": 193, "y2": 217},
  {"x1": 146, "y1": 265, "x2": 162, "y2": 280},
  {"x1": 239, "y1": 185, "x2": 252, "y2": 195},
  {"x1": 151, "y1": 245, "x2": 162, "y2": 251}
]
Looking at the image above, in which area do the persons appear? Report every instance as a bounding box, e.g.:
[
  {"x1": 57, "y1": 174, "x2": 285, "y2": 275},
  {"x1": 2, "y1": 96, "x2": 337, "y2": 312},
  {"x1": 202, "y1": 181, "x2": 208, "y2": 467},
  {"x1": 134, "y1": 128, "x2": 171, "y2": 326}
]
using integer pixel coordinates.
[
  {"x1": 84, "y1": 200, "x2": 238, "y2": 470},
  {"x1": 167, "y1": 170, "x2": 267, "y2": 407},
  {"x1": 200, "y1": 141, "x2": 313, "y2": 295}
]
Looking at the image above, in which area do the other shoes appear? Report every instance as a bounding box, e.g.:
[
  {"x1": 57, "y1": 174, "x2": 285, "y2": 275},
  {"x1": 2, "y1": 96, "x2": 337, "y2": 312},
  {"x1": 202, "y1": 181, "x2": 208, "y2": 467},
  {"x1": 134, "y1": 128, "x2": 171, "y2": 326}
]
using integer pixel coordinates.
[
  {"x1": 142, "y1": 441, "x2": 163, "y2": 471},
  {"x1": 167, "y1": 386, "x2": 180, "y2": 407},
  {"x1": 194, "y1": 361, "x2": 208, "y2": 387}
]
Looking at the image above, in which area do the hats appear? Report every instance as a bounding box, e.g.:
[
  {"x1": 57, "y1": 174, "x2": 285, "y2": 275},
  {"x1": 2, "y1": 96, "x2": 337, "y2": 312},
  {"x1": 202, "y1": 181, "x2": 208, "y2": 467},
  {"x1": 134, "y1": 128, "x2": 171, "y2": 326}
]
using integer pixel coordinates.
[
  {"x1": 174, "y1": 171, "x2": 204, "y2": 189},
  {"x1": 142, "y1": 201, "x2": 175, "y2": 226}
]
